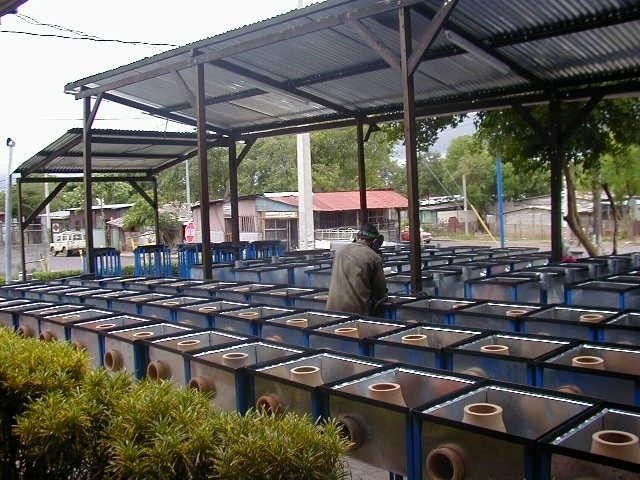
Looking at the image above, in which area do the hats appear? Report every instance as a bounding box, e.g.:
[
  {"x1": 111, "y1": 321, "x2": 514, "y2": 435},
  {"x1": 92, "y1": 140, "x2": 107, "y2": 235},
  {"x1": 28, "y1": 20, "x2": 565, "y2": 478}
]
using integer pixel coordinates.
[{"x1": 357, "y1": 222, "x2": 377, "y2": 238}]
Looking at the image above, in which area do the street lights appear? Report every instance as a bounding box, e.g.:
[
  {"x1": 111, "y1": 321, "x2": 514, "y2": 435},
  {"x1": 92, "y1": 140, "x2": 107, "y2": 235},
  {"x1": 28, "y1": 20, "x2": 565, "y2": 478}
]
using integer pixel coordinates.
[{"x1": 5, "y1": 138, "x2": 15, "y2": 284}]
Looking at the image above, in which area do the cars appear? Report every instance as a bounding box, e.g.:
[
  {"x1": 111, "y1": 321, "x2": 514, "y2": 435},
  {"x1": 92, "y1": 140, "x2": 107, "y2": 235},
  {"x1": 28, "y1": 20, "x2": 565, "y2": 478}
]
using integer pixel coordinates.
[{"x1": 401, "y1": 225, "x2": 433, "y2": 244}]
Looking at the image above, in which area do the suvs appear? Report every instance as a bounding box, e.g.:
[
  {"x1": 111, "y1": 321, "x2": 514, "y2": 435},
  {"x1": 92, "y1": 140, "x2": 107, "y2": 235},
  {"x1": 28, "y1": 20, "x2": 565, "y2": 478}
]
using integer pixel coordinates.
[{"x1": 49, "y1": 231, "x2": 86, "y2": 256}]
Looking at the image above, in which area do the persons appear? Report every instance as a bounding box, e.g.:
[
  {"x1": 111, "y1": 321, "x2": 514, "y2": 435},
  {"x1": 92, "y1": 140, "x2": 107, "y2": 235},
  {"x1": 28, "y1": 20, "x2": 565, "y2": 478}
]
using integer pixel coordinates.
[{"x1": 326, "y1": 223, "x2": 386, "y2": 316}]
